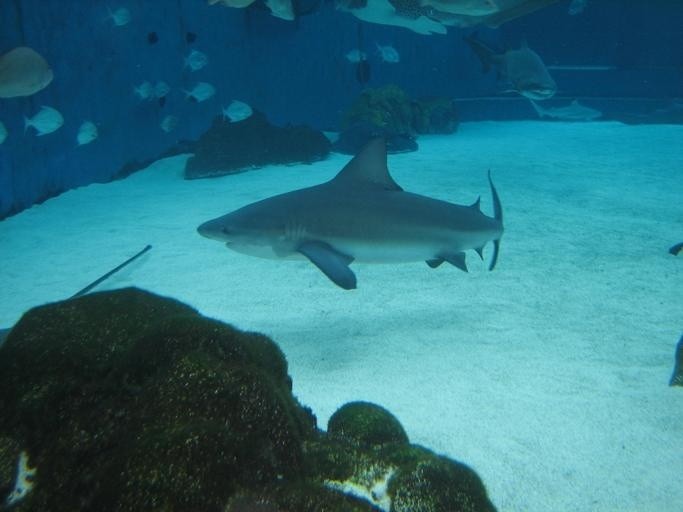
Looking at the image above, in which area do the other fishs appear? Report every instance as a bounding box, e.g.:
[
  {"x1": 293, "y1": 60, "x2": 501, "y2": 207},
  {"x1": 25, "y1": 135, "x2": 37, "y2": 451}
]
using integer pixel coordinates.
[
  {"x1": 2, "y1": 3, "x2": 447, "y2": 150},
  {"x1": 1, "y1": 1, "x2": 606, "y2": 297}
]
